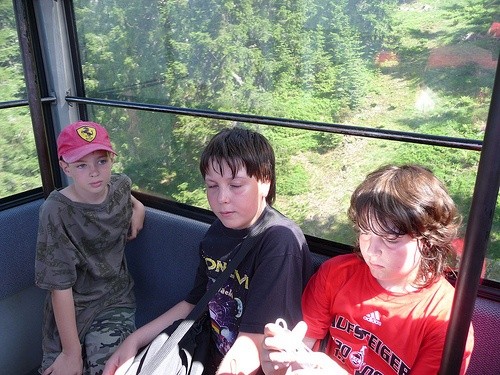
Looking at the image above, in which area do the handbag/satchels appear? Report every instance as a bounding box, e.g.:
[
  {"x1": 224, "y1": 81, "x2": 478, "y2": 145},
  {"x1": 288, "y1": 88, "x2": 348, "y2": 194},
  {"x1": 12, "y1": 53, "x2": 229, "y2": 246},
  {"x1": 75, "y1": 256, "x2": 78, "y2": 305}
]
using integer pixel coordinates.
[{"x1": 112, "y1": 306, "x2": 215, "y2": 375}]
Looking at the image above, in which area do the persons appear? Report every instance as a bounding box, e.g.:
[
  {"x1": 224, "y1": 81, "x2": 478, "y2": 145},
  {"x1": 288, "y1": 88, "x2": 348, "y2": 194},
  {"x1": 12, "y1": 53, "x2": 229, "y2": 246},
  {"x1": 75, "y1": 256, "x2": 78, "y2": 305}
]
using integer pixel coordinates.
[
  {"x1": 31, "y1": 120, "x2": 146, "y2": 375},
  {"x1": 96, "y1": 125, "x2": 310, "y2": 375},
  {"x1": 255, "y1": 164, "x2": 475, "y2": 374}
]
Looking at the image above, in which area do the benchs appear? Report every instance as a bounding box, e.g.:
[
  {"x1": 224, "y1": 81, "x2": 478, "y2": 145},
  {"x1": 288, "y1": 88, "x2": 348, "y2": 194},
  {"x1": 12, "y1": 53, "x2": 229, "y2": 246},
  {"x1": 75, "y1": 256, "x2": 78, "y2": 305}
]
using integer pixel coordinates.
[
  {"x1": 1, "y1": 198, "x2": 47, "y2": 375},
  {"x1": 126, "y1": 206, "x2": 500, "y2": 375}
]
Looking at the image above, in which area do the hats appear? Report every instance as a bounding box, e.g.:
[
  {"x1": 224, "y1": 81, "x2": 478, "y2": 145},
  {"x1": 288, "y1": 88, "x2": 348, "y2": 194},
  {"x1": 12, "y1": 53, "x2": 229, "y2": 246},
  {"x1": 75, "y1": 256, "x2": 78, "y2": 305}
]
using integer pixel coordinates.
[{"x1": 57, "y1": 120, "x2": 119, "y2": 162}]
